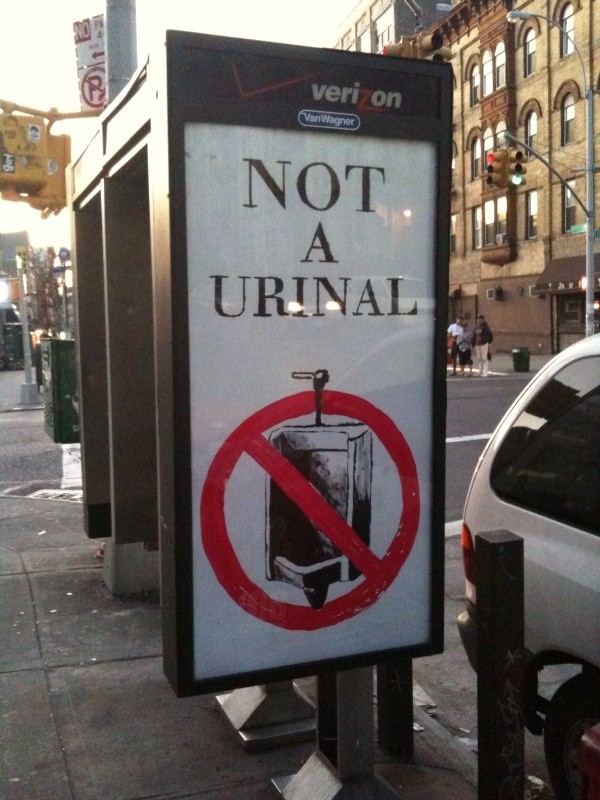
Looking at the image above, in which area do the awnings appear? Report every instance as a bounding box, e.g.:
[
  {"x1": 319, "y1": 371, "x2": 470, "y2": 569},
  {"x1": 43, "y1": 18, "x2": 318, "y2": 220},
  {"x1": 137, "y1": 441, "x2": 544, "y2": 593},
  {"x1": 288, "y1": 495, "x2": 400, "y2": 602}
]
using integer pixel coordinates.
[{"x1": 536, "y1": 253, "x2": 600, "y2": 292}]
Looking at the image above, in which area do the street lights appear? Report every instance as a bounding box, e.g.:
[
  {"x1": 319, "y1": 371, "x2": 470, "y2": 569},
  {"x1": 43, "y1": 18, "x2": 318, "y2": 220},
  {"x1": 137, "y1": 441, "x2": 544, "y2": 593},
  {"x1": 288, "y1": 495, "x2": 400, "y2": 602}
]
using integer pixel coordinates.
[{"x1": 506, "y1": 10, "x2": 596, "y2": 338}]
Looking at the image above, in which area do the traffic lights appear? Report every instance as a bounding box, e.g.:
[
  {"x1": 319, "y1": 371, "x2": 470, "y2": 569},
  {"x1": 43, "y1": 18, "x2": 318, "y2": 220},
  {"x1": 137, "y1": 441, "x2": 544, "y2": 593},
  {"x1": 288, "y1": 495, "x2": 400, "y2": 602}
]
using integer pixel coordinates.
[
  {"x1": 486, "y1": 147, "x2": 506, "y2": 189},
  {"x1": 504, "y1": 147, "x2": 525, "y2": 189},
  {"x1": 520, "y1": 150, "x2": 528, "y2": 186}
]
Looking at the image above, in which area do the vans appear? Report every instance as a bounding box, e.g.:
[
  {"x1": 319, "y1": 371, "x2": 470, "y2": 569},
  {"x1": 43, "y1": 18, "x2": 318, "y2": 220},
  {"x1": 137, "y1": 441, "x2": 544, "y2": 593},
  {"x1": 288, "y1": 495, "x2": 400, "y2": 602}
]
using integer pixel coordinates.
[{"x1": 0, "y1": 302, "x2": 35, "y2": 371}]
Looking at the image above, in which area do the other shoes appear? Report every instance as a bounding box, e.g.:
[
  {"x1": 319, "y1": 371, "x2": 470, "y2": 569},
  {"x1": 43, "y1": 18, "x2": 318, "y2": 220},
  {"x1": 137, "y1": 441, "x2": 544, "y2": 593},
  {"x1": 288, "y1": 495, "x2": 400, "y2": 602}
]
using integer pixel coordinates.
[
  {"x1": 449, "y1": 372, "x2": 457, "y2": 376},
  {"x1": 458, "y1": 373, "x2": 464, "y2": 377}
]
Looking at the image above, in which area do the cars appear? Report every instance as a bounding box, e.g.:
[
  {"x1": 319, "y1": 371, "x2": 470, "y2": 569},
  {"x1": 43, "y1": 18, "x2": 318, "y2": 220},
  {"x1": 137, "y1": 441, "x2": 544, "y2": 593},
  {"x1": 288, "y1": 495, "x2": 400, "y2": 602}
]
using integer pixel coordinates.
[{"x1": 453, "y1": 328, "x2": 600, "y2": 800}]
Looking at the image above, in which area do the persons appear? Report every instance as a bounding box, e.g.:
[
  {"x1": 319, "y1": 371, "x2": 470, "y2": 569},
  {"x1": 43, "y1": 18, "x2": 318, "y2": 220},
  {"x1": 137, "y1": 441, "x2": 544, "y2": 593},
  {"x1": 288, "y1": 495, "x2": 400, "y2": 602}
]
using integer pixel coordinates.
[
  {"x1": 446, "y1": 316, "x2": 465, "y2": 377},
  {"x1": 471, "y1": 314, "x2": 493, "y2": 378},
  {"x1": 456, "y1": 323, "x2": 473, "y2": 377}
]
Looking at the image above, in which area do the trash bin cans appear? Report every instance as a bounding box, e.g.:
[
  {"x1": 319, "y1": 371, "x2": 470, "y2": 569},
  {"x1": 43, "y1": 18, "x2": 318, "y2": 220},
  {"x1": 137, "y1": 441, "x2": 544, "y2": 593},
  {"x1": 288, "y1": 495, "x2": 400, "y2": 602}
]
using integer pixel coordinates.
[{"x1": 512, "y1": 347, "x2": 530, "y2": 372}]
